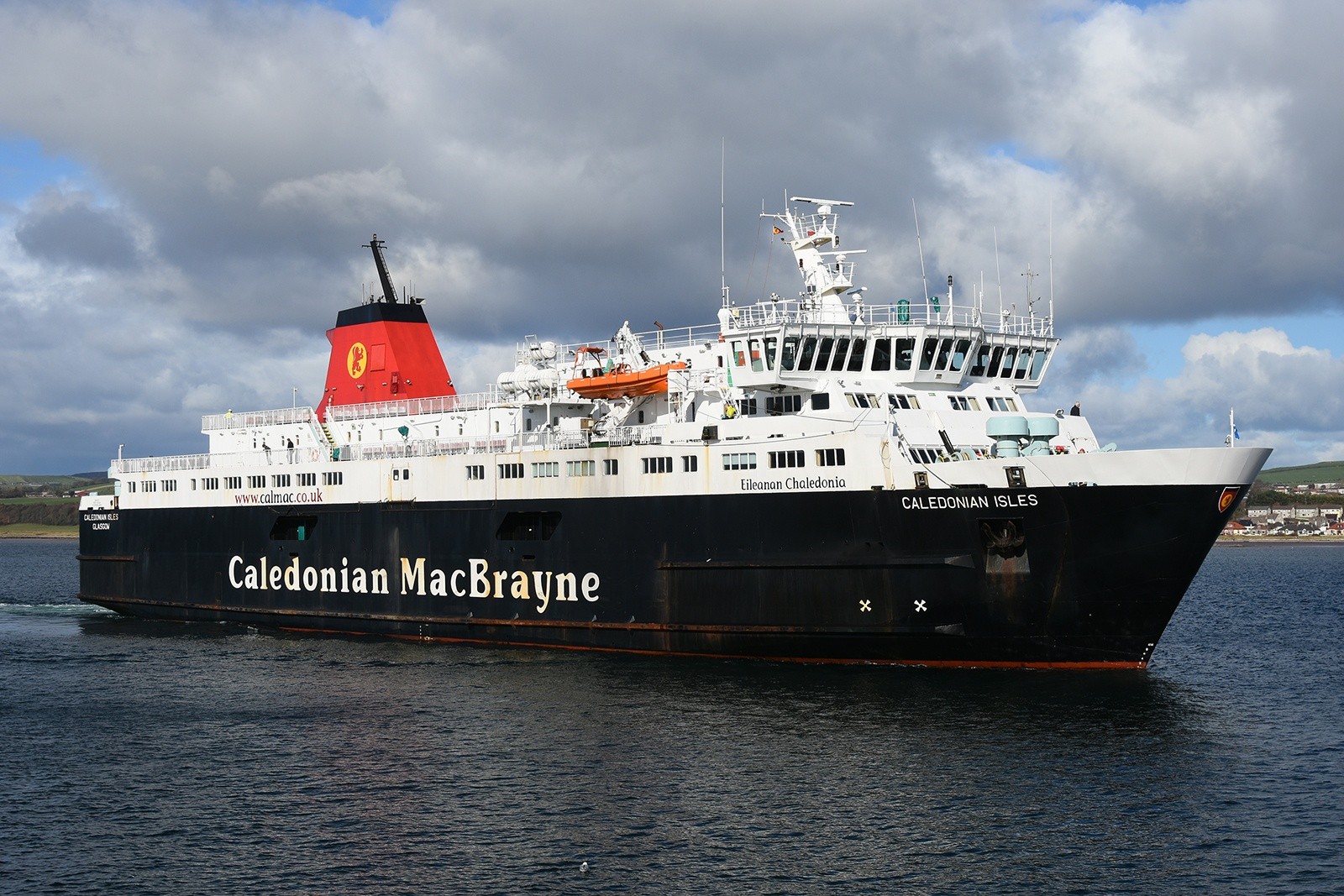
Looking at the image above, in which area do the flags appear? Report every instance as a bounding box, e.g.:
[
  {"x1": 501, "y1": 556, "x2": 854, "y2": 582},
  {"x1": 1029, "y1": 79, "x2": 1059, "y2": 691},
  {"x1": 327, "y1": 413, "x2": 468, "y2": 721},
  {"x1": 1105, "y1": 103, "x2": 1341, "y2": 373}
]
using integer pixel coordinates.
[{"x1": 773, "y1": 226, "x2": 784, "y2": 234}]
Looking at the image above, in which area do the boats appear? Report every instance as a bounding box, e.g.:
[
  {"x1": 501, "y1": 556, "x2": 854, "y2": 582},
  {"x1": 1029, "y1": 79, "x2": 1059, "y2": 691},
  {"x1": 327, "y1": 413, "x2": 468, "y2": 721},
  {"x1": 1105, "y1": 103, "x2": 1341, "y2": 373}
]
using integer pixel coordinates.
[
  {"x1": 566, "y1": 359, "x2": 687, "y2": 401},
  {"x1": 75, "y1": 133, "x2": 1276, "y2": 704}
]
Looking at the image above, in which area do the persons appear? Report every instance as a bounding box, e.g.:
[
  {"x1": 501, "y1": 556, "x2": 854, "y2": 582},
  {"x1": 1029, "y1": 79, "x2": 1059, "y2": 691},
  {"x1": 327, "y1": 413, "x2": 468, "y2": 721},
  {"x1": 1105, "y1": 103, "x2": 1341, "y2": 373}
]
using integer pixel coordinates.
[
  {"x1": 1070, "y1": 402, "x2": 1082, "y2": 414},
  {"x1": 262, "y1": 444, "x2": 272, "y2": 465},
  {"x1": 286, "y1": 438, "x2": 295, "y2": 464},
  {"x1": 725, "y1": 400, "x2": 737, "y2": 418},
  {"x1": 770, "y1": 402, "x2": 783, "y2": 415}
]
